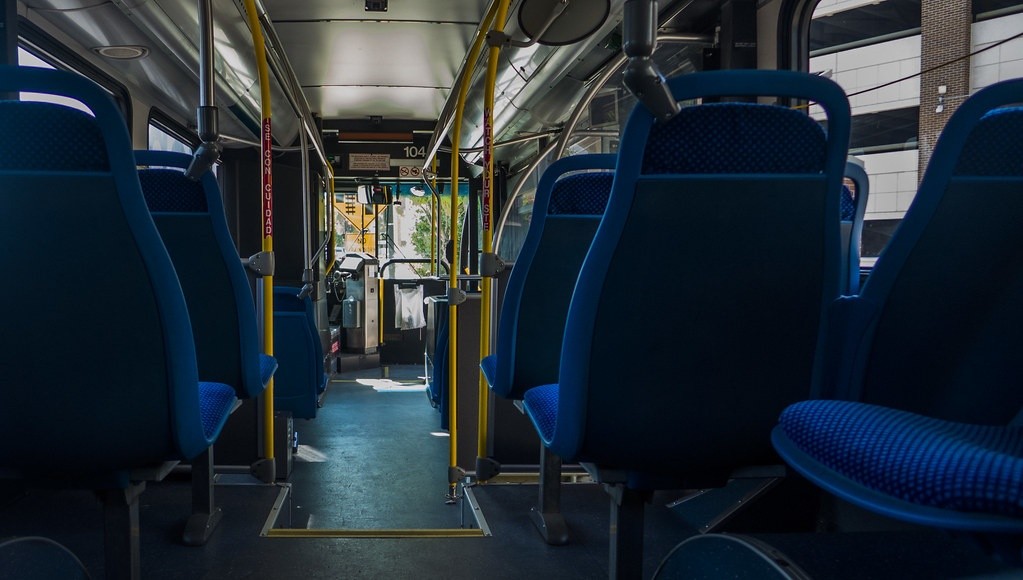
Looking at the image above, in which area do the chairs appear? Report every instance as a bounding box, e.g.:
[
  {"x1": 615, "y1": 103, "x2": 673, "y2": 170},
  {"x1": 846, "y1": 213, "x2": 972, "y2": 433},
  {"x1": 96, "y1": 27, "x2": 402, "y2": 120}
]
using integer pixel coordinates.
[
  {"x1": 480, "y1": 69, "x2": 1023, "y2": 580},
  {"x1": 0, "y1": 65, "x2": 333, "y2": 580}
]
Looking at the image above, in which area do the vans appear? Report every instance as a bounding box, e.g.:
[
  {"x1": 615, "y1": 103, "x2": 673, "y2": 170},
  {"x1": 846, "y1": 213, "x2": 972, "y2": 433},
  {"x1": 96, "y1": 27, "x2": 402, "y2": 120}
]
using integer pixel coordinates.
[{"x1": 325, "y1": 247, "x2": 346, "y2": 260}]
